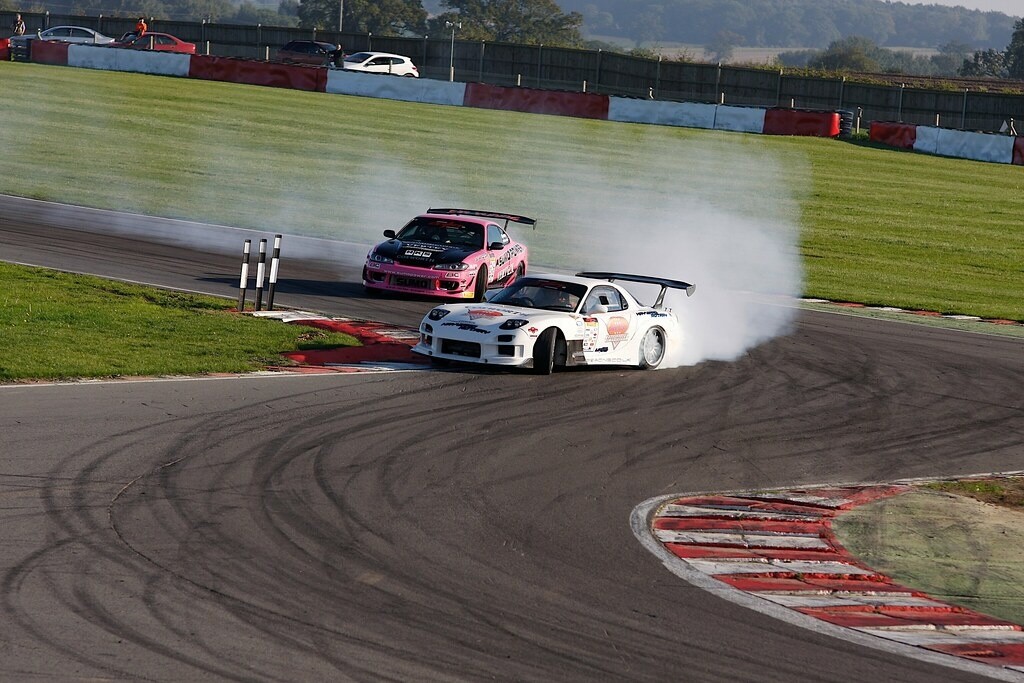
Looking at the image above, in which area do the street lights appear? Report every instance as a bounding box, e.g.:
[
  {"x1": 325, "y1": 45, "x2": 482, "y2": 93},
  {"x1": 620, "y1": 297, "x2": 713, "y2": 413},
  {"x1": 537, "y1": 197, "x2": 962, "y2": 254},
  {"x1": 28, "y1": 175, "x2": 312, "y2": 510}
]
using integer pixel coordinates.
[{"x1": 446, "y1": 20, "x2": 462, "y2": 80}]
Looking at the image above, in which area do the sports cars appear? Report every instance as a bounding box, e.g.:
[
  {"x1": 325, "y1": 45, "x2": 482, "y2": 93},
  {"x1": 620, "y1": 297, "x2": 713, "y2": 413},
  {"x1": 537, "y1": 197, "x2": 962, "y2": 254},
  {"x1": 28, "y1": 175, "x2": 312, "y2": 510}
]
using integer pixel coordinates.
[
  {"x1": 410, "y1": 270, "x2": 695, "y2": 375},
  {"x1": 361, "y1": 206, "x2": 540, "y2": 301}
]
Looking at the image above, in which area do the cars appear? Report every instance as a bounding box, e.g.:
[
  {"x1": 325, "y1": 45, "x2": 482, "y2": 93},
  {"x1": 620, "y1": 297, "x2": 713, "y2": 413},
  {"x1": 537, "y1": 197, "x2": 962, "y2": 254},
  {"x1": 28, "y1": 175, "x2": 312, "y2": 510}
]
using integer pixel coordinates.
[
  {"x1": 110, "y1": 31, "x2": 197, "y2": 53},
  {"x1": 10, "y1": 27, "x2": 116, "y2": 46}
]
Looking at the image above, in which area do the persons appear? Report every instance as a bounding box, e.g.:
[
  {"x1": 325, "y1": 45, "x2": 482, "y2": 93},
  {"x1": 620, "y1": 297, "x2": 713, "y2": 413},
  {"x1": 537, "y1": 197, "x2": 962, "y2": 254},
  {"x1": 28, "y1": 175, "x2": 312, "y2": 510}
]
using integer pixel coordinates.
[
  {"x1": 326, "y1": 44, "x2": 345, "y2": 68},
  {"x1": 135, "y1": 17, "x2": 147, "y2": 37},
  {"x1": 10, "y1": 13, "x2": 25, "y2": 36}
]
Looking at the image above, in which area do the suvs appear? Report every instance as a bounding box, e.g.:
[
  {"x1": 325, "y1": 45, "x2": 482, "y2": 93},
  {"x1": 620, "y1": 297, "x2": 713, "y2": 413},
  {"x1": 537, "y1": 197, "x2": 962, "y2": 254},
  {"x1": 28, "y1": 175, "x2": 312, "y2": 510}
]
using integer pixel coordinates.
[
  {"x1": 328, "y1": 52, "x2": 420, "y2": 78},
  {"x1": 276, "y1": 40, "x2": 351, "y2": 65}
]
